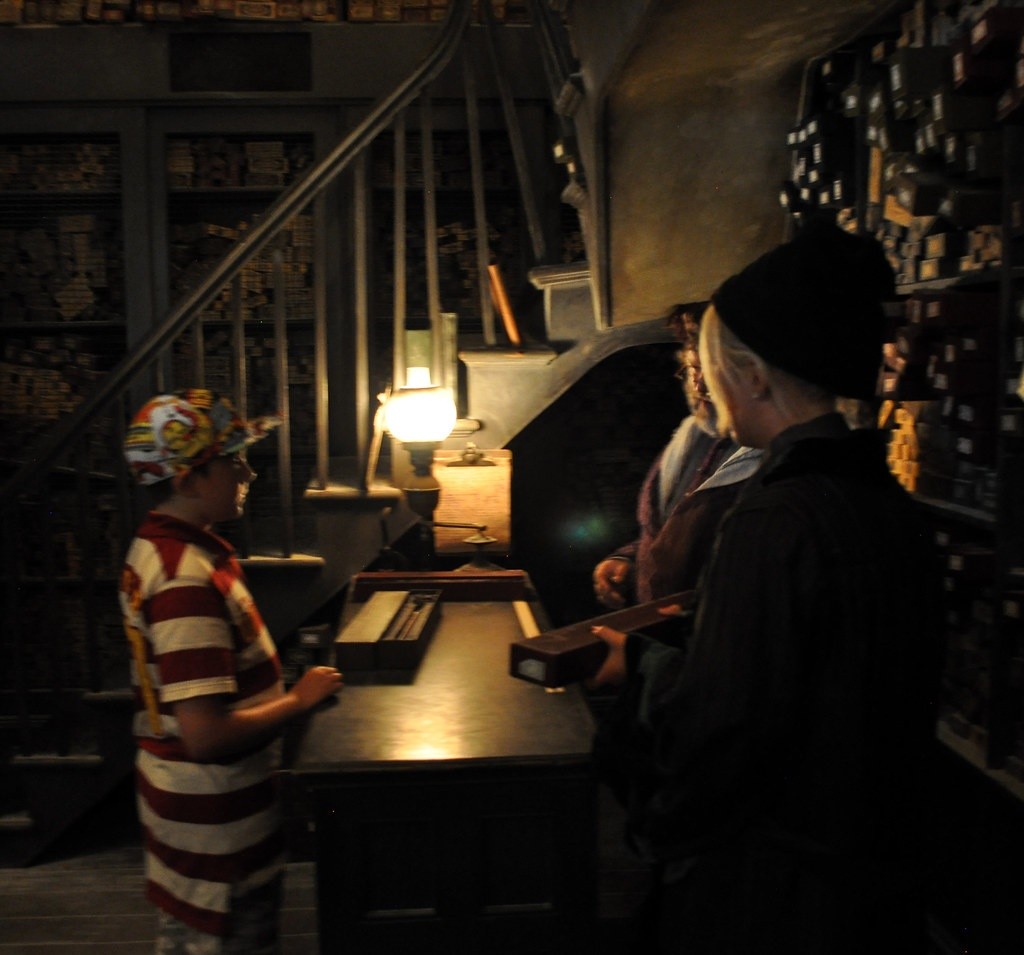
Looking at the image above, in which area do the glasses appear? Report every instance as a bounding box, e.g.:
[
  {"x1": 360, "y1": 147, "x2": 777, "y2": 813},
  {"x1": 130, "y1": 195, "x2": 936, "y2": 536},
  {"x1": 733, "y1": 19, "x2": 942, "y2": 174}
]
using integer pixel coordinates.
[{"x1": 675, "y1": 365, "x2": 710, "y2": 401}]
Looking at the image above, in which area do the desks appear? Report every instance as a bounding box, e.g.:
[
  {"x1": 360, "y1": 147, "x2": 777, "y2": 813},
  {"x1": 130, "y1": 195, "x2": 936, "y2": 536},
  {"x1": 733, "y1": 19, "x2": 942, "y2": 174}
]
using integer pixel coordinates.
[{"x1": 293, "y1": 574, "x2": 602, "y2": 954}]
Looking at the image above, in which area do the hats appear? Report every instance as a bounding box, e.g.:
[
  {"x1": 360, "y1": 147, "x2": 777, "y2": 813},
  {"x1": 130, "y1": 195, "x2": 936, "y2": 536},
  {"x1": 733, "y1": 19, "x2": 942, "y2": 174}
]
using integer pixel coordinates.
[
  {"x1": 121, "y1": 389, "x2": 286, "y2": 485},
  {"x1": 711, "y1": 232, "x2": 895, "y2": 402}
]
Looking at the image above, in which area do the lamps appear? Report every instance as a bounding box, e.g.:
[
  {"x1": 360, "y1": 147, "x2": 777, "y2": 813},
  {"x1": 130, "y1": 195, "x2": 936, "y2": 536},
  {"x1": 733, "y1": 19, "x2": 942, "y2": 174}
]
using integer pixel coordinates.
[{"x1": 384, "y1": 366, "x2": 501, "y2": 574}]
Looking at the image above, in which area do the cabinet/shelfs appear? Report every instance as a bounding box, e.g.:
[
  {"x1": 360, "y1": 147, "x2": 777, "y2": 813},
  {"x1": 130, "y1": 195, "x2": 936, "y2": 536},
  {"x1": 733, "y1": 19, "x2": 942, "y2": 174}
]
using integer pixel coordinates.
[
  {"x1": 0, "y1": 17, "x2": 568, "y2": 727},
  {"x1": 783, "y1": 0, "x2": 1024, "y2": 955}
]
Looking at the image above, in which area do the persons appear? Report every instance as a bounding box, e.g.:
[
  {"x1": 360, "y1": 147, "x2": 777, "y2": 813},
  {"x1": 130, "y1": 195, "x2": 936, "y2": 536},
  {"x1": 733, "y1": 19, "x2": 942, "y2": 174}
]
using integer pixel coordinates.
[
  {"x1": 587, "y1": 260, "x2": 945, "y2": 955},
  {"x1": 119, "y1": 388, "x2": 345, "y2": 955},
  {"x1": 592, "y1": 299, "x2": 741, "y2": 611}
]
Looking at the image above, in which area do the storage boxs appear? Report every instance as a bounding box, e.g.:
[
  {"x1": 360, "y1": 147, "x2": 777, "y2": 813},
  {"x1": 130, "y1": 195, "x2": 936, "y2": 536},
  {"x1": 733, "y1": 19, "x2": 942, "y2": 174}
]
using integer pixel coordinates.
[
  {"x1": 511, "y1": 590, "x2": 696, "y2": 689},
  {"x1": 334, "y1": 585, "x2": 442, "y2": 673}
]
